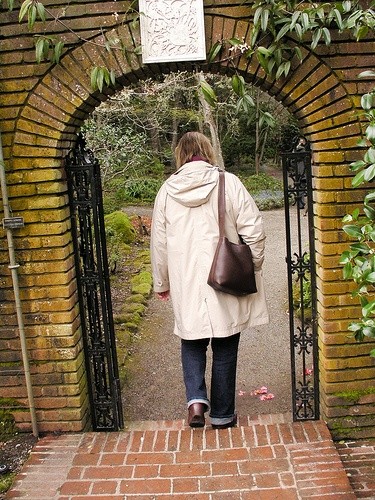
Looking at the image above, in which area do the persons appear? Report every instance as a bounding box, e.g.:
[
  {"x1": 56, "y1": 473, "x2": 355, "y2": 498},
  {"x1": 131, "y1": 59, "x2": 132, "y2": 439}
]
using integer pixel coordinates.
[{"x1": 150, "y1": 133, "x2": 271, "y2": 429}]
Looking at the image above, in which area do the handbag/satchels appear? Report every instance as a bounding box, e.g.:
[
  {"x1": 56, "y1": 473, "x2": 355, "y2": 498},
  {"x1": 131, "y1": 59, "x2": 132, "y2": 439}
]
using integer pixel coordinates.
[{"x1": 207, "y1": 236, "x2": 258, "y2": 296}]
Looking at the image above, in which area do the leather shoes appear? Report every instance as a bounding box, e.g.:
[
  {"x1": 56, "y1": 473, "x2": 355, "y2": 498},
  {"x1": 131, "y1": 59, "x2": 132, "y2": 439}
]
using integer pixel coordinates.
[
  {"x1": 187, "y1": 403, "x2": 206, "y2": 427},
  {"x1": 211, "y1": 415, "x2": 238, "y2": 430}
]
